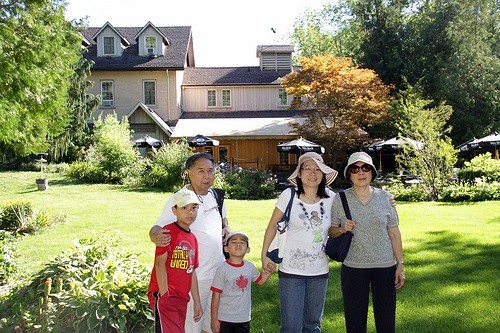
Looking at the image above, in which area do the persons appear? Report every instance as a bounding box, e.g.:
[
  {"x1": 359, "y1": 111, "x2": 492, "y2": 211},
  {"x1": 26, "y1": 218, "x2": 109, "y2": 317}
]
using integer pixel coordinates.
[
  {"x1": 210, "y1": 231, "x2": 276, "y2": 333},
  {"x1": 261, "y1": 152, "x2": 355, "y2": 333},
  {"x1": 328, "y1": 152, "x2": 405, "y2": 333},
  {"x1": 149, "y1": 152, "x2": 232, "y2": 333},
  {"x1": 147, "y1": 189, "x2": 204, "y2": 333}
]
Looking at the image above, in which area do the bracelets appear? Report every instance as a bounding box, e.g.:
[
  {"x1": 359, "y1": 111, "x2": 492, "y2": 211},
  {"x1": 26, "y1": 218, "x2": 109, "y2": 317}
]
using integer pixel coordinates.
[{"x1": 397, "y1": 261, "x2": 405, "y2": 264}]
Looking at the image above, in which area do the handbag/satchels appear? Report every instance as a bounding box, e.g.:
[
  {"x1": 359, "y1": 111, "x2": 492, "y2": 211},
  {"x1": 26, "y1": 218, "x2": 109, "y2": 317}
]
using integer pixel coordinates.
[
  {"x1": 266, "y1": 187, "x2": 295, "y2": 263},
  {"x1": 325, "y1": 191, "x2": 354, "y2": 262}
]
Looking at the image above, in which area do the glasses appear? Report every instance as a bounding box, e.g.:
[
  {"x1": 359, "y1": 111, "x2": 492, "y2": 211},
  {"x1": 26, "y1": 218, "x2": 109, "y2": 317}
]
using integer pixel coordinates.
[
  {"x1": 348, "y1": 163, "x2": 373, "y2": 173},
  {"x1": 301, "y1": 167, "x2": 322, "y2": 172}
]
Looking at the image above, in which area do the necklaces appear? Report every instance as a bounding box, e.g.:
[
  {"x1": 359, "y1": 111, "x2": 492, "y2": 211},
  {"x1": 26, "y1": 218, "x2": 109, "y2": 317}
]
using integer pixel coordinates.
[{"x1": 297, "y1": 193, "x2": 324, "y2": 232}]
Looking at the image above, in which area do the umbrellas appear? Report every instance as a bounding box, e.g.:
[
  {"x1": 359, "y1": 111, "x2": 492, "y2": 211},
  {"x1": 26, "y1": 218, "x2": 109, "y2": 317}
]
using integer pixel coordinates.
[
  {"x1": 279, "y1": 136, "x2": 325, "y2": 156},
  {"x1": 190, "y1": 134, "x2": 220, "y2": 153},
  {"x1": 470, "y1": 133, "x2": 500, "y2": 157},
  {"x1": 372, "y1": 133, "x2": 424, "y2": 174},
  {"x1": 132, "y1": 135, "x2": 162, "y2": 158}
]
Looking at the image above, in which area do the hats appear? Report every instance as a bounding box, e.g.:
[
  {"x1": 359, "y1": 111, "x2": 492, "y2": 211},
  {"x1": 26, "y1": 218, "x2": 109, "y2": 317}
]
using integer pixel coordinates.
[
  {"x1": 172, "y1": 190, "x2": 200, "y2": 208},
  {"x1": 224, "y1": 230, "x2": 249, "y2": 245},
  {"x1": 287, "y1": 152, "x2": 339, "y2": 187},
  {"x1": 344, "y1": 151, "x2": 379, "y2": 182}
]
